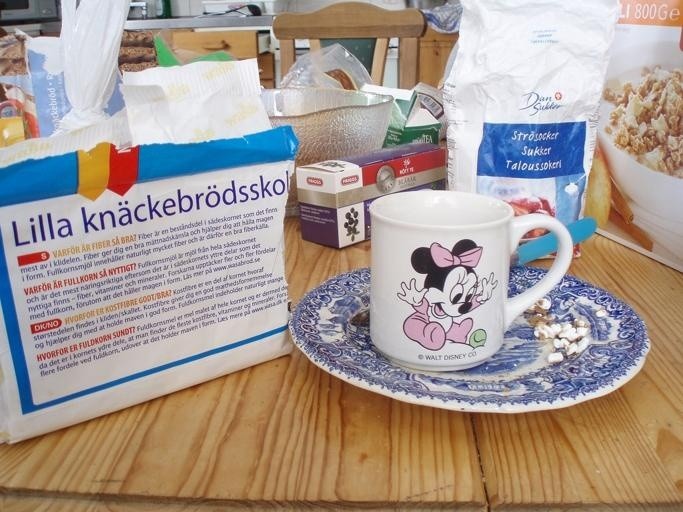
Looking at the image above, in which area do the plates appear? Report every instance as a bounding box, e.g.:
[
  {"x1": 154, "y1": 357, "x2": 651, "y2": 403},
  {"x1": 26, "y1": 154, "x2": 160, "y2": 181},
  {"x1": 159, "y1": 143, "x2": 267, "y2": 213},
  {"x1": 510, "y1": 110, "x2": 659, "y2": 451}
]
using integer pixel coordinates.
[{"x1": 291, "y1": 256, "x2": 653, "y2": 413}]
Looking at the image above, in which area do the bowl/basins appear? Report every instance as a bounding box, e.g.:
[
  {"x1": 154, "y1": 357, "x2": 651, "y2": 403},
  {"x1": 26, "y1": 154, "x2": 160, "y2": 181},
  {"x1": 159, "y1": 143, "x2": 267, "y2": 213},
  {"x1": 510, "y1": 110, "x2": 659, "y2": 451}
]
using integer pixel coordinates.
[
  {"x1": 598, "y1": 40, "x2": 683, "y2": 221},
  {"x1": 256, "y1": 88, "x2": 396, "y2": 215}
]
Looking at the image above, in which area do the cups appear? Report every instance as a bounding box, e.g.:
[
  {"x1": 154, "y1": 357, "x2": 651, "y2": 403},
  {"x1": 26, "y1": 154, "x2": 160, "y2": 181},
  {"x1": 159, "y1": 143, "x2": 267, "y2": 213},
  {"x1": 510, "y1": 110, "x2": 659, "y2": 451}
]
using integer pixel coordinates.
[{"x1": 365, "y1": 190, "x2": 574, "y2": 374}]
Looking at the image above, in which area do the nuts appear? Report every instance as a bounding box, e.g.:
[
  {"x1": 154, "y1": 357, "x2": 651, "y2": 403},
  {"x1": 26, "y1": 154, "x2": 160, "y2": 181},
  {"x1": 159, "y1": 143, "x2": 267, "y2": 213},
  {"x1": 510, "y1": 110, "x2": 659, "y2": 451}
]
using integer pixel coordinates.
[{"x1": 604, "y1": 66, "x2": 683, "y2": 180}]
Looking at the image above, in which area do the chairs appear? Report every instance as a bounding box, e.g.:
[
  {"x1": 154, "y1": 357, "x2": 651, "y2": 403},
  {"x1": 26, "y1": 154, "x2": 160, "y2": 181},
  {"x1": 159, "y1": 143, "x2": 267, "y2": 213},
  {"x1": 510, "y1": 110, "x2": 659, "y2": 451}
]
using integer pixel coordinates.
[{"x1": 273, "y1": 2, "x2": 426, "y2": 90}]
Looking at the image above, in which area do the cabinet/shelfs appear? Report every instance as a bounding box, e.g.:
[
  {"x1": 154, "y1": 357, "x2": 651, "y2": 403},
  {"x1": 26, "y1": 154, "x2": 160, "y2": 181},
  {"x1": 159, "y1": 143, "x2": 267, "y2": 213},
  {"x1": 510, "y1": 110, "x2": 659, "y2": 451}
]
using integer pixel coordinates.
[{"x1": 173, "y1": 26, "x2": 275, "y2": 90}]
